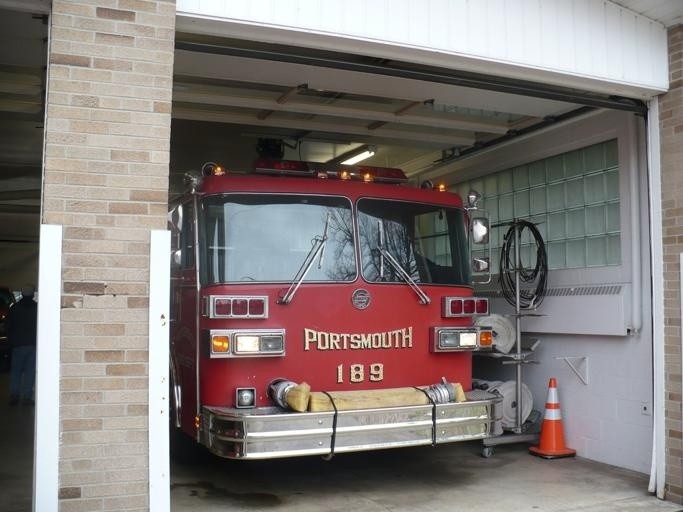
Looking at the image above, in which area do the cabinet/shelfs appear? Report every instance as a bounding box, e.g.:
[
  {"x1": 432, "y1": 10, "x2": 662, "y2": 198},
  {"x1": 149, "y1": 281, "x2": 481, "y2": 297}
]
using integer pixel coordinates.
[{"x1": 471, "y1": 216, "x2": 550, "y2": 458}]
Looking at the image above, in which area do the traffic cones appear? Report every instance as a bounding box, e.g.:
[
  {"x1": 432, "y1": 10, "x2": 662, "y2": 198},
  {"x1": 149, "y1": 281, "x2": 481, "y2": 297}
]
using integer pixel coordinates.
[{"x1": 528, "y1": 375, "x2": 577, "y2": 464}]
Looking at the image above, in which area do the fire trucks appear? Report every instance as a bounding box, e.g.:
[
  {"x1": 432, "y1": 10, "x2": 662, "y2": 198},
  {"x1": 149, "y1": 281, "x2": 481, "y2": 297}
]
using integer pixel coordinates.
[{"x1": 169, "y1": 159, "x2": 503, "y2": 463}]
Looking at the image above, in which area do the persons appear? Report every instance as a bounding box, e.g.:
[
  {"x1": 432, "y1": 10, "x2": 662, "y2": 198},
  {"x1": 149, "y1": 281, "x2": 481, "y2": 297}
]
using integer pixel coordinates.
[{"x1": 4, "y1": 283, "x2": 37, "y2": 404}]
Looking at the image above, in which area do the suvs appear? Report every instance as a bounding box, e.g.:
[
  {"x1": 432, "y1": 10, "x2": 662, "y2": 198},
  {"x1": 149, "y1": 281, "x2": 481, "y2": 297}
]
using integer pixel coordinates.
[{"x1": 0, "y1": 286, "x2": 17, "y2": 371}]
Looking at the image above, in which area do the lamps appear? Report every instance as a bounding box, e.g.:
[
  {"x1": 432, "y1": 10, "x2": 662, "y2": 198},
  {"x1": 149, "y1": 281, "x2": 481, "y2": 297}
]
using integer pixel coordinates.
[{"x1": 324, "y1": 144, "x2": 377, "y2": 166}]
